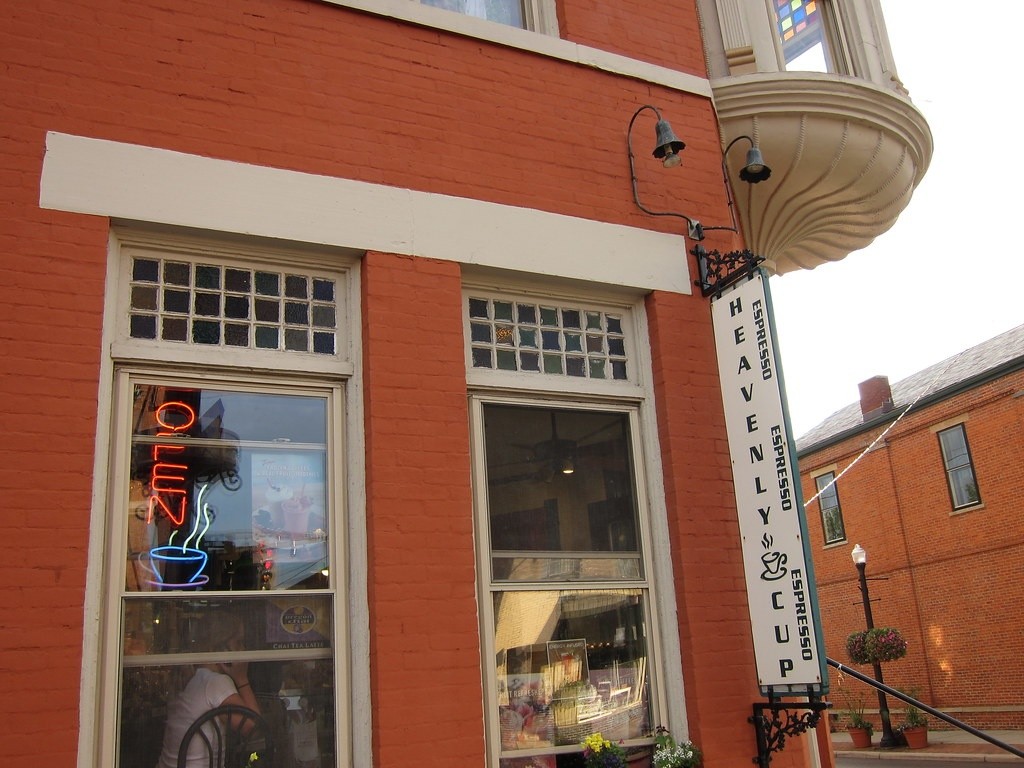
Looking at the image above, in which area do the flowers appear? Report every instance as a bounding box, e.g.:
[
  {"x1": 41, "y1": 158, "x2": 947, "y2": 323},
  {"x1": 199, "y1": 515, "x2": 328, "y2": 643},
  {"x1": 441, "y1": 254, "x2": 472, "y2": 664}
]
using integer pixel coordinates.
[
  {"x1": 844, "y1": 627, "x2": 907, "y2": 665},
  {"x1": 580, "y1": 732, "x2": 626, "y2": 768},
  {"x1": 652, "y1": 723, "x2": 704, "y2": 768}
]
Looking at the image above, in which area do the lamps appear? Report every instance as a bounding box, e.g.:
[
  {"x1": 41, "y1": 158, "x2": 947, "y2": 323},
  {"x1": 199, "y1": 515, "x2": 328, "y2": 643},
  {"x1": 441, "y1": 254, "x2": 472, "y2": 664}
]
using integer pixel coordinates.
[
  {"x1": 697, "y1": 136, "x2": 772, "y2": 240},
  {"x1": 627, "y1": 105, "x2": 701, "y2": 241}
]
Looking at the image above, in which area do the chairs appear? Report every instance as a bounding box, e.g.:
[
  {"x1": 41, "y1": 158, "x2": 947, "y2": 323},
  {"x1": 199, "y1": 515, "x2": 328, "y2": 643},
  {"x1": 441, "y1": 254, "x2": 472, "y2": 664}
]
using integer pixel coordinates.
[{"x1": 176, "y1": 704, "x2": 275, "y2": 768}]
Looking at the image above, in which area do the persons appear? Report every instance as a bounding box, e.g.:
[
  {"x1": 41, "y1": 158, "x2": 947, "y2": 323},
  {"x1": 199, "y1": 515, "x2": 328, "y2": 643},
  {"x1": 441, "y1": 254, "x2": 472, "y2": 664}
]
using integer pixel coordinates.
[{"x1": 155, "y1": 610, "x2": 260, "y2": 768}]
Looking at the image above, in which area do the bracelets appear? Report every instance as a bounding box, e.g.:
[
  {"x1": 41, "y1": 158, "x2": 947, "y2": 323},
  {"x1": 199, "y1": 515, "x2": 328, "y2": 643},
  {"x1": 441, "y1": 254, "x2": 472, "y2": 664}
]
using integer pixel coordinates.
[{"x1": 238, "y1": 683, "x2": 250, "y2": 689}]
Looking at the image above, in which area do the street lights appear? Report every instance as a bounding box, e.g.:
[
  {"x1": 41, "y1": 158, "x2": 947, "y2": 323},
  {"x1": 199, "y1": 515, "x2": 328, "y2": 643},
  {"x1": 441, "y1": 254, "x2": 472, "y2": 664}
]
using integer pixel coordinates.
[{"x1": 851, "y1": 544, "x2": 898, "y2": 749}]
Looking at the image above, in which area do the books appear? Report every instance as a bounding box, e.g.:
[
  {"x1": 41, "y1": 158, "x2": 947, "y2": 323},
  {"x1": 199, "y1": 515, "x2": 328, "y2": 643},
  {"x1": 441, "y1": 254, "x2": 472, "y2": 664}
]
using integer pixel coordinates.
[{"x1": 604, "y1": 657, "x2": 646, "y2": 704}]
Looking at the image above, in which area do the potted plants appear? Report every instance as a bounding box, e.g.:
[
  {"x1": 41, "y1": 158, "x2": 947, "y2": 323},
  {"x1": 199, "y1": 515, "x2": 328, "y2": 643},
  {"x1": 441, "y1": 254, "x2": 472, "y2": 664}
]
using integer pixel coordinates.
[
  {"x1": 833, "y1": 686, "x2": 877, "y2": 748},
  {"x1": 893, "y1": 686, "x2": 927, "y2": 749}
]
